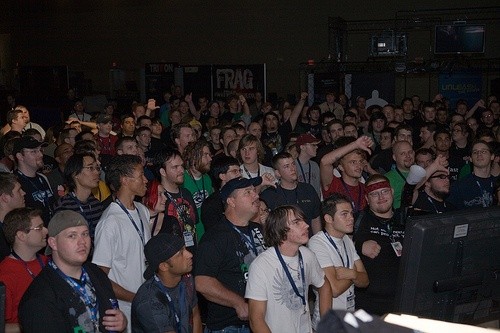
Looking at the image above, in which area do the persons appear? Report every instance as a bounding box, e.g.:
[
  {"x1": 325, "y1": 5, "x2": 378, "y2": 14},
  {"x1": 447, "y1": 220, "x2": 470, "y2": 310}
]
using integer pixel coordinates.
[{"x1": 0, "y1": 86, "x2": 500, "y2": 333}]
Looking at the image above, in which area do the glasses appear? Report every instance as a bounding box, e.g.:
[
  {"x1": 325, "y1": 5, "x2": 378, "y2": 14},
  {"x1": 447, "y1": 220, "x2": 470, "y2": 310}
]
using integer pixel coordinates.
[
  {"x1": 82, "y1": 166, "x2": 100, "y2": 174},
  {"x1": 20, "y1": 223, "x2": 45, "y2": 233},
  {"x1": 483, "y1": 115, "x2": 493, "y2": 118},
  {"x1": 430, "y1": 174, "x2": 451, "y2": 179},
  {"x1": 368, "y1": 189, "x2": 391, "y2": 198},
  {"x1": 452, "y1": 129, "x2": 464, "y2": 132},
  {"x1": 23, "y1": 148, "x2": 45, "y2": 155}
]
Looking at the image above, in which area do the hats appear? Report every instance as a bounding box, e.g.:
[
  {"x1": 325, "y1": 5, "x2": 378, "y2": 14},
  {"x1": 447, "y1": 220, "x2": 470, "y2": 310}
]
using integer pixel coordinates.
[
  {"x1": 143, "y1": 233, "x2": 185, "y2": 280},
  {"x1": 190, "y1": 120, "x2": 202, "y2": 128},
  {"x1": 220, "y1": 176, "x2": 263, "y2": 199},
  {"x1": 295, "y1": 134, "x2": 321, "y2": 147},
  {"x1": 45, "y1": 211, "x2": 88, "y2": 256},
  {"x1": 96, "y1": 114, "x2": 114, "y2": 124},
  {"x1": 13, "y1": 136, "x2": 49, "y2": 156}
]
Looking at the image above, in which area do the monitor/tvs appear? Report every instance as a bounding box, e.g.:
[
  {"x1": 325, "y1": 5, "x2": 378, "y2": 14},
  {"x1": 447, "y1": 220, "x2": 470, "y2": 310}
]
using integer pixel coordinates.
[{"x1": 393, "y1": 207, "x2": 500, "y2": 324}]
